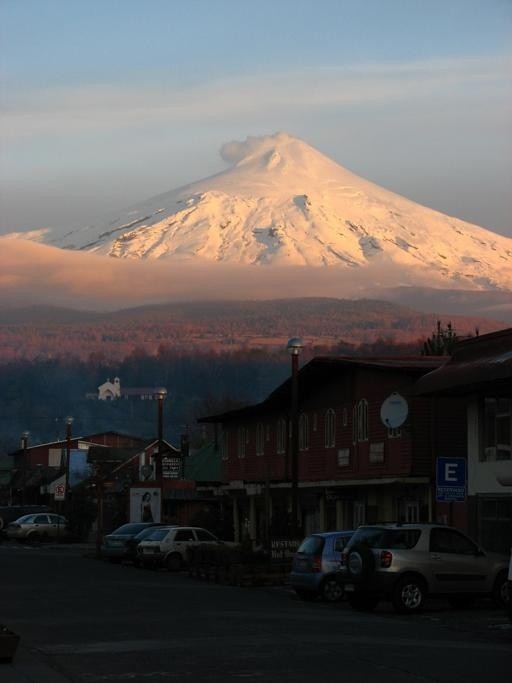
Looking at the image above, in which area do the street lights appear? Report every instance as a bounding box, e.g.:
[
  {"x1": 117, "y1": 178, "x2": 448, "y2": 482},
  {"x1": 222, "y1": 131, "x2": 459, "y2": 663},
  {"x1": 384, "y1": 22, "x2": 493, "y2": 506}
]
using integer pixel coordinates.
[
  {"x1": 154, "y1": 387, "x2": 168, "y2": 481},
  {"x1": 286, "y1": 337, "x2": 303, "y2": 538},
  {"x1": 21, "y1": 430, "x2": 29, "y2": 468},
  {"x1": 65, "y1": 415, "x2": 75, "y2": 503}
]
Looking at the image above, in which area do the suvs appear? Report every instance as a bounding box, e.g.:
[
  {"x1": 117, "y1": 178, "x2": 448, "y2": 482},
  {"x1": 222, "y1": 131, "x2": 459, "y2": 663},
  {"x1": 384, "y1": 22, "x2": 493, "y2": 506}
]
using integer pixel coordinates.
[{"x1": 336, "y1": 520, "x2": 512, "y2": 615}]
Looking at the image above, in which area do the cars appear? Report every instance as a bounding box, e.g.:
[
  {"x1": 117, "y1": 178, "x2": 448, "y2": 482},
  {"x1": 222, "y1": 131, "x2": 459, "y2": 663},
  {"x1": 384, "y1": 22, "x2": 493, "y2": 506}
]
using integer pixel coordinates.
[
  {"x1": 288, "y1": 531, "x2": 356, "y2": 603},
  {"x1": 100, "y1": 522, "x2": 244, "y2": 572},
  {"x1": 6, "y1": 513, "x2": 71, "y2": 546}
]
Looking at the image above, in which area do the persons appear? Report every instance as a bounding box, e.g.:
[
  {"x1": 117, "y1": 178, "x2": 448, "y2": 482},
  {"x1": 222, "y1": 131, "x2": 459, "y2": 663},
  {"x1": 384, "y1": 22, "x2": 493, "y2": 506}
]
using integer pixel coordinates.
[
  {"x1": 507, "y1": 547, "x2": 512, "y2": 624},
  {"x1": 140, "y1": 492, "x2": 153, "y2": 522}
]
